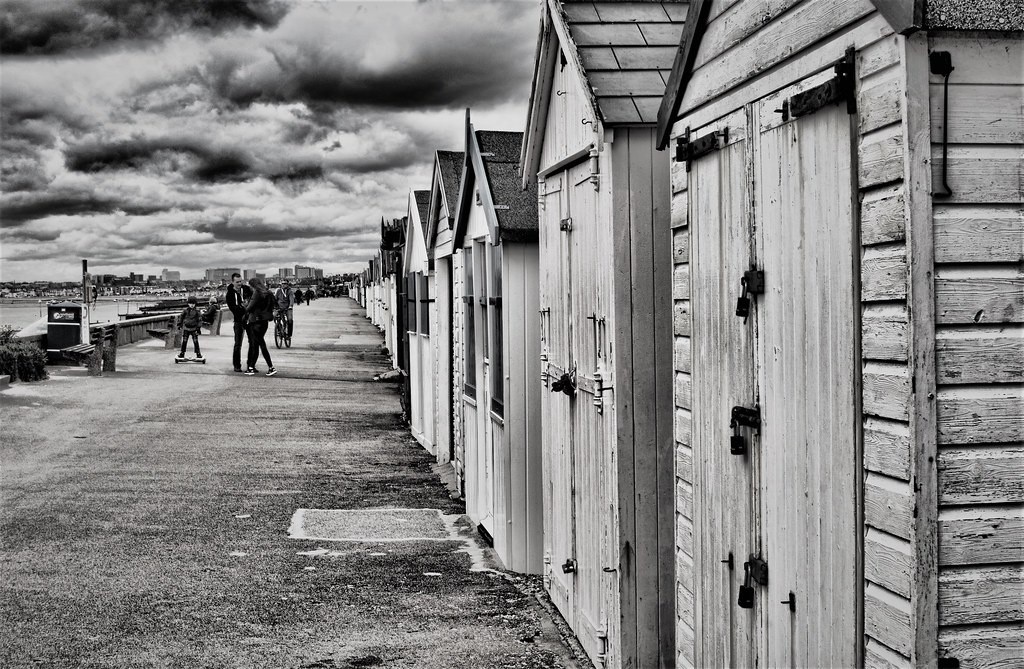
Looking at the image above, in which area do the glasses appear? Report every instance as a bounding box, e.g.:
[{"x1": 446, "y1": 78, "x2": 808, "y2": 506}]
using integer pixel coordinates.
[
  {"x1": 282, "y1": 283, "x2": 287, "y2": 285},
  {"x1": 236, "y1": 280, "x2": 241, "y2": 283}
]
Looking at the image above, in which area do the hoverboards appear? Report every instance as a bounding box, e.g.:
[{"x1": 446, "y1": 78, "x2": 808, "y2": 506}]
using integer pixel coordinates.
[{"x1": 174, "y1": 355, "x2": 207, "y2": 364}]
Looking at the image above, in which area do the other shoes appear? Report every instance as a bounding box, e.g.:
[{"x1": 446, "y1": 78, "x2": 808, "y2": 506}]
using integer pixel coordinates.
[
  {"x1": 196, "y1": 354, "x2": 202, "y2": 358},
  {"x1": 179, "y1": 352, "x2": 184, "y2": 358},
  {"x1": 234, "y1": 367, "x2": 242, "y2": 372},
  {"x1": 254, "y1": 369, "x2": 259, "y2": 374}
]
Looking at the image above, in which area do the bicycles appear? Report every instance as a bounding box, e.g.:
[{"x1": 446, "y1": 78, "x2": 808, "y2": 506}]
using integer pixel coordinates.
[{"x1": 273, "y1": 307, "x2": 294, "y2": 348}]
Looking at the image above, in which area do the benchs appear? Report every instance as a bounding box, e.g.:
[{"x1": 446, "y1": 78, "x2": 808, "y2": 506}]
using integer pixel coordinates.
[
  {"x1": 146, "y1": 315, "x2": 183, "y2": 350},
  {"x1": 60, "y1": 324, "x2": 120, "y2": 376},
  {"x1": 202, "y1": 310, "x2": 223, "y2": 336}
]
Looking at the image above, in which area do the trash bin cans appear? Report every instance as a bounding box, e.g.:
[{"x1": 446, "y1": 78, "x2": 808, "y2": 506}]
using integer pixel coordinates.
[{"x1": 47, "y1": 302, "x2": 82, "y2": 366}]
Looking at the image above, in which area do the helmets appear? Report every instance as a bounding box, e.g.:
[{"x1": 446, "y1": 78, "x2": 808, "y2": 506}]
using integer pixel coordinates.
[{"x1": 187, "y1": 296, "x2": 198, "y2": 303}]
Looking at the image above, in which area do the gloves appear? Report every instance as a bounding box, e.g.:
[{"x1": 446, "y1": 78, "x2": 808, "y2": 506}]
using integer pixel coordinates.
[
  {"x1": 178, "y1": 325, "x2": 180, "y2": 330},
  {"x1": 197, "y1": 329, "x2": 201, "y2": 335}
]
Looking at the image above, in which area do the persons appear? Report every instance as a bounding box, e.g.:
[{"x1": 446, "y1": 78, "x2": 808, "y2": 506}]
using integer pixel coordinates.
[
  {"x1": 294, "y1": 287, "x2": 302, "y2": 305},
  {"x1": 201, "y1": 297, "x2": 217, "y2": 325},
  {"x1": 225, "y1": 273, "x2": 276, "y2": 376},
  {"x1": 273, "y1": 279, "x2": 294, "y2": 338},
  {"x1": 304, "y1": 287, "x2": 312, "y2": 305},
  {"x1": 178, "y1": 296, "x2": 203, "y2": 358}
]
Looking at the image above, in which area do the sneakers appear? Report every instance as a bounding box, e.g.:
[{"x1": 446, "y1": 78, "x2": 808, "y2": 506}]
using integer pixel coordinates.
[
  {"x1": 244, "y1": 368, "x2": 255, "y2": 375},
  {"x1": 266, "y1": 368, "x2": 277, "y2": 376}
]
